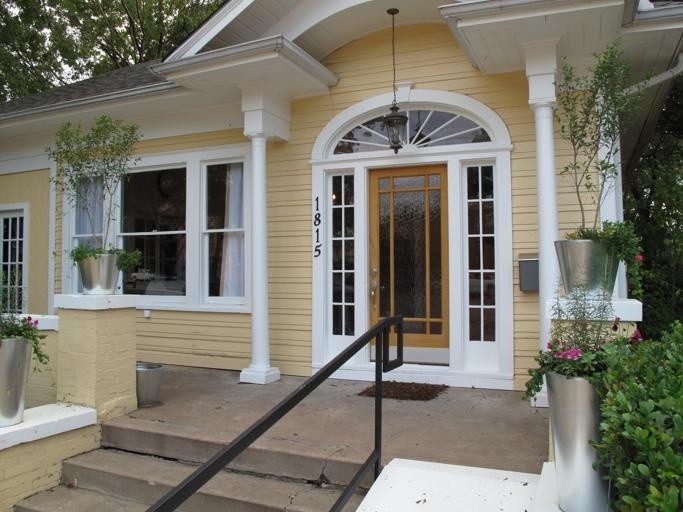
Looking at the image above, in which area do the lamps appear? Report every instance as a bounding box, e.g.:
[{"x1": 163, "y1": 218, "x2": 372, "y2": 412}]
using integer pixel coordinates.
[{"x1": 381, "y1": 7, "x2": 409, "y2": 155}]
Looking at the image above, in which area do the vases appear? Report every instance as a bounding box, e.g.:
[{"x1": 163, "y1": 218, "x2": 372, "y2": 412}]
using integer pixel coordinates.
[
  {"x1": 544, "y1": 369, "x2": 616, "y2": 512},
  {"x1": 1, "y1": 337, "x2": 34, "y2": 427}
]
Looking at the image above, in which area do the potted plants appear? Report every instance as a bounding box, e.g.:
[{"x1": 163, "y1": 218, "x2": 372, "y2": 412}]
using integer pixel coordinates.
[
  {"x1": 550, "y1": 31, "x2": 656, "y2": 302},
  {"x1": 43, "y1": 111, "x2": 144, "y2": 295}
]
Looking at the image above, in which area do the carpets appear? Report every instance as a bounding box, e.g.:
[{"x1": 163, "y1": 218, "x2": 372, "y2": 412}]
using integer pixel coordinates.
[{"x1": 358, "y1": 379, "x2": 448, "y2": 401}]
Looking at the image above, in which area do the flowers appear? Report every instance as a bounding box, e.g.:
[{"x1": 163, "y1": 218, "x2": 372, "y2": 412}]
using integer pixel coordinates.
[
  {"x1": 0, "y1": 309, "x2": 52, "y2": 377},
  {"x1": 520, "y1": 280, "x2": 643, "y2": 415}
]
